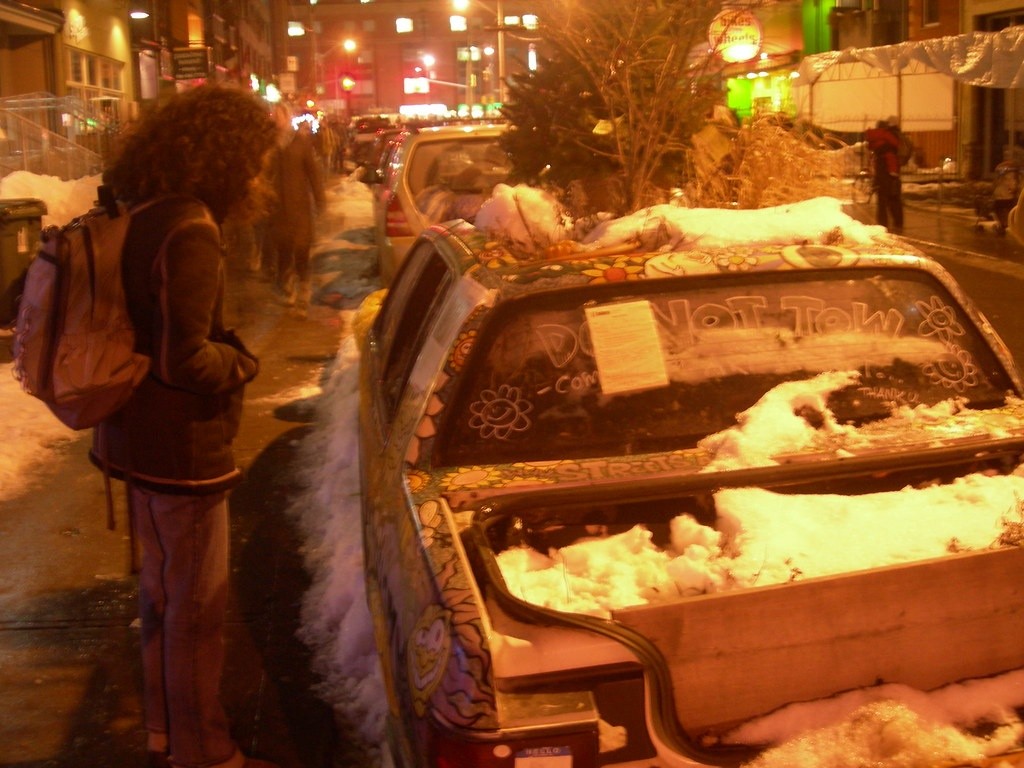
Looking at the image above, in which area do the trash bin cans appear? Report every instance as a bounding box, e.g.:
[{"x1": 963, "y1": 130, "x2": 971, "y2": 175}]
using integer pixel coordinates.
[{"x1": 0, "y1": 197, "x2": 48, "y2": 328}]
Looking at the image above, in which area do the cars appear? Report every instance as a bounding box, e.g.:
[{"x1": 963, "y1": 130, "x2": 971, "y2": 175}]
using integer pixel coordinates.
[
  {"x1": 352, "y1": 125, "x2": 565, "y2": 289},
  {"x1": 340, "y1": 118, "x2": 419, "y2": 185},
  {"x1": 340, "y1": 218, "x2": 1024, "y2": 768}
]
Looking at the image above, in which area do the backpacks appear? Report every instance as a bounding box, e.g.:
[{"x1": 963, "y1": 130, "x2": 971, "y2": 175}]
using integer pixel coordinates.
[{"x1": 13, "y1": 182, "x2": 185, "y2": 431}]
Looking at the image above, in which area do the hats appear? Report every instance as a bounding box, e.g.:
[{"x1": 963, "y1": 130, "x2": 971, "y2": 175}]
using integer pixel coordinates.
[{"x1": 888, "y1": 115, "x2": 899, "y2": 127}]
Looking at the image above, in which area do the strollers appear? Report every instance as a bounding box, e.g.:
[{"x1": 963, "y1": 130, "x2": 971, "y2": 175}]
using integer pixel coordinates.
[{"x1": 967, "y1": 159, "x2": 1024, "y2": 237}]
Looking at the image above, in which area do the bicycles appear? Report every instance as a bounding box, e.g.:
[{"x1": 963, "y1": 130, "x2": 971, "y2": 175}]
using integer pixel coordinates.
[{"x1": 849, "y1": 167, "x2": 875, "y2": 206}]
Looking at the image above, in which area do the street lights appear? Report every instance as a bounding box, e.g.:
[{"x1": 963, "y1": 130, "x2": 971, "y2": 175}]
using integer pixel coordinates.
[
  {"x1": 420, "y1": 52, "x2": 475, "y2": 120},
  {"x1": 308, "y1": 38, "x2": 358, "y2": 109},
  {"x1": 451, "y1": 0, "x2": 507, "y2": 105}
]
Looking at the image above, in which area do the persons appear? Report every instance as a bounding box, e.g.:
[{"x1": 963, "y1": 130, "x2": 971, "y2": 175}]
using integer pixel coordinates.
[
  {"x1": 257, "y1": 101, "x2": 354, "y2": 318},
  {"x1": 86, "y1": 84, "x2": 279, "y2": 768},
  {"x1": 864, "y1": 117, "x2": 906, "y2": 234}
]
